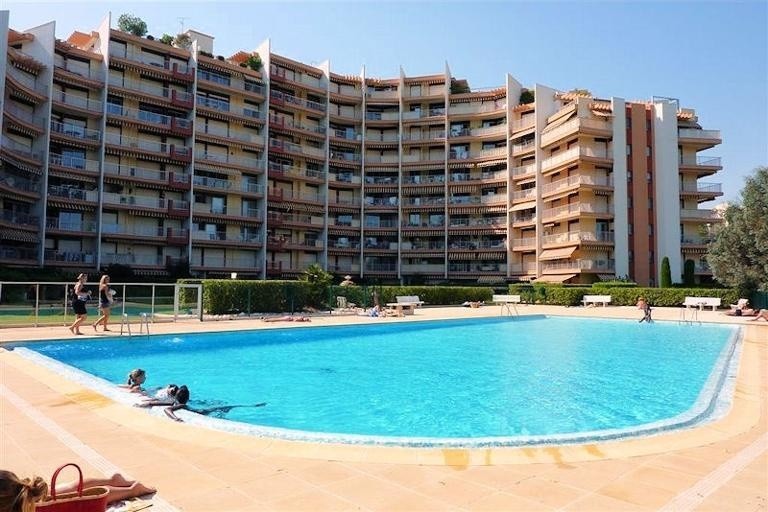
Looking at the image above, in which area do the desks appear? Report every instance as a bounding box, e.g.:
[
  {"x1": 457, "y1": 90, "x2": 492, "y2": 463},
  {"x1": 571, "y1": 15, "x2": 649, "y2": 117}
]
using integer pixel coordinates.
[{"x1": 387, "y1": 303, "x2": 416, "y2": 318}]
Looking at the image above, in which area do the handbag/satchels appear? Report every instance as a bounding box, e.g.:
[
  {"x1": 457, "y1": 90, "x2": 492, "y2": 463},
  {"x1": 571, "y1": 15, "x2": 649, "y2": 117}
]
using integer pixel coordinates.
[{"x1": 35, "y1": 464, "x2": 110, "y2": 512}]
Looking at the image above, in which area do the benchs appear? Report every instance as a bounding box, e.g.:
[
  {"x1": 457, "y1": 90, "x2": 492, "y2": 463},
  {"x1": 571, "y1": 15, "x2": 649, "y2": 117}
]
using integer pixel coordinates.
[
  {"x1": 580, "y1": 295, "x2": 611, "y2": 307},
  {"x1": 682, "y1": 296, "x2": 722, "y2": 311},
  {"x1": 492, "y1": 295, "x2": 521, "y2": 306},
  {"x1": 396, "y1": 295, "x2": 424, "y2": 309}
]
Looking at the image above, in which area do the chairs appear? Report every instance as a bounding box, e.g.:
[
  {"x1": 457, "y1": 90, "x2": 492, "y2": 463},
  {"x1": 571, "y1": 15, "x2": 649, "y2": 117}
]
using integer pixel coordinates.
[
  {"x1": 729, "y1": 299, "x2": 749, "y2": 311},
  {"x1": 337, "y1": 296, "x2": 357, "y2": 314}
]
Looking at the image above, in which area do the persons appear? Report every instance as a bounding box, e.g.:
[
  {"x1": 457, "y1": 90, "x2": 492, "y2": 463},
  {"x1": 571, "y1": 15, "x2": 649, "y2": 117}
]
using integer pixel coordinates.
[
  {"x1": 128, "y1": 369, "x2": 146, "y2": 393},
  {"x1": 139, "y1": 385, "x2": 189, "y2": 421},
  {"x1": 264, "y1": 316, "x2": 309, "y2": 322},
  {"x1": 47, "y1": 473, "x2": 157, "y2": 506},
  {"x1": 69, "y1": 273, "x2": 91, "y2": 335},
  {"x1": 371, "y1": 305, "x2": 380, "y2": 317},
  {"x1": 93, "y1": 275, "x2": 113, "y2": 331},
  {"x1": 725, "y1": 307, "x2": 768, "y2": 322},
  {"x1": 636, "y1": 298, "x2": 652, "y2": 323},
  {"x1": 0, "y1": 470, "x2": 49, "y2": 512},
  {"x1": 142, "y1": 383, "x2": 178, "y2": 400}
]
